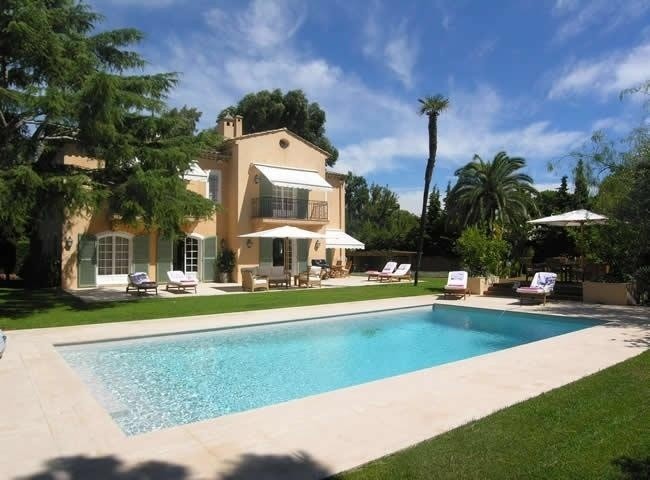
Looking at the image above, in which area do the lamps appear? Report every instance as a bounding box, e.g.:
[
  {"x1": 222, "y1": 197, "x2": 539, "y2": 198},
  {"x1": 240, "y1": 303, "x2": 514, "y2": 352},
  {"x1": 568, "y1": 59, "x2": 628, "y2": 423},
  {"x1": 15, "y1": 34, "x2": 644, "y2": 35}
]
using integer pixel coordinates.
[
  {"x1": 315, "y1": 239, "x2": 321, "y2": 249},
  {"x1": 246, "y1": 238, "x2": 253, "y2": 248}
]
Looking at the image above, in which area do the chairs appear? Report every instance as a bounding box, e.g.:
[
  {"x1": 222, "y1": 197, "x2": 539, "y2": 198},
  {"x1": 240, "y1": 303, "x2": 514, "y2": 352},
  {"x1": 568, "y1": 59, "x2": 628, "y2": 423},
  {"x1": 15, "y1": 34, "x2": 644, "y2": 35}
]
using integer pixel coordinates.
[
  {"x1": 125, "y1": 259, "x2": 413, "y2": 297},
  {"x1": 442, "y1": 256, "x2": 585, "y2": 306}
]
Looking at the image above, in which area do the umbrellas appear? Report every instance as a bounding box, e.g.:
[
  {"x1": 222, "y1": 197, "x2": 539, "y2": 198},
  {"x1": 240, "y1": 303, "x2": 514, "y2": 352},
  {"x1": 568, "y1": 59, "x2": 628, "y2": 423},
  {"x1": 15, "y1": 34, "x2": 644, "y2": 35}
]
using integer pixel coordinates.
[
  {"x1": 235, "y1": 225, "x2": 340, "y2": 287},
  {"x1": 526, "y1": 208, "x2": 631, "y2": 283}
]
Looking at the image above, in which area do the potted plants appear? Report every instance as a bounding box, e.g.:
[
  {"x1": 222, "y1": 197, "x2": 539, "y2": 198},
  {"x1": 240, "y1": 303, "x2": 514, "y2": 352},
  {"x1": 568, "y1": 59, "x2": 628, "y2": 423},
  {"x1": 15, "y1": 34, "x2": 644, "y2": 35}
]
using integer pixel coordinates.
[{"x1": 215, "y1": 248, "x2": 238, "y2": 283}]
